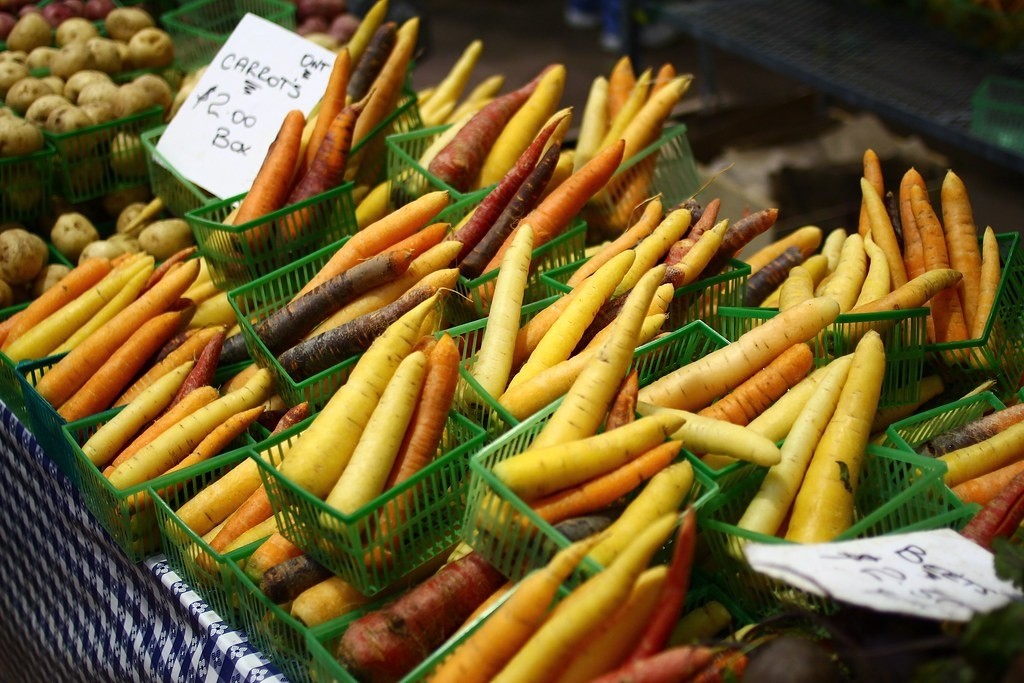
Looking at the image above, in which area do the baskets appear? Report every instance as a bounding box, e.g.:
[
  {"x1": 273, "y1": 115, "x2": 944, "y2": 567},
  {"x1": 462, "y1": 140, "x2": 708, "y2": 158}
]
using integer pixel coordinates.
[
  {"x1": 463, "y1": 395, "x2": 717, "y2": 586},
  {"x1": 584, "y1": 119, "x2": 698, "y2": 236},
  {"x1": 715, "y1": 302, "x2": 929, "y2": 410},
  {"x1": 1, "y1": 244, "x2": 575, "y2": 683},
  {"x1": 540, "y1": 239, "x2": 751, "y2": 334},
  {"x1": 185, "y1": 179, "x2": 357, "y2": 301},
  {"x1": 693, "y1": 388, "x2": 1015, "y2": 625},
  {"x1": 387, "y1": 123, "x2": 491, "y2": 209},
  {"x1": 0, "y1": 0, "x2": 429, "y2": 221},
  {"x1": 227, "y1": 235, "x2": 373, "y2": 413},
  {"x1": 926, "y1": 230, "x2": 1024, "y2": 398},
  {"x1": 451, "y1": 219, "x2": 585, "y2": 321},
  {"x1": 248, "y1": 395, "x2": 488, "y2": 593},
  {"x1": 623, "y1": 319, "x2": 795, "y2": 484}
]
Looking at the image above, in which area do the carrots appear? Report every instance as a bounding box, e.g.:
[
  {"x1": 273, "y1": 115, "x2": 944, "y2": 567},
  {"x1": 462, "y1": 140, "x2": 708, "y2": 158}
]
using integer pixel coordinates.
[
  {"x1": 0, "y1": 244, "x2": 776, "y2": 683},
  {"x1": 207, "y1": 0, "x2": 1024, "y2": 583}
]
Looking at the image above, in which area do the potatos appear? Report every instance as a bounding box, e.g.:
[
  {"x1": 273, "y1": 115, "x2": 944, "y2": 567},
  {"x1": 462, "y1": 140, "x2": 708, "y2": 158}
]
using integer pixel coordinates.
[{"x1": 0, "y1": 0, "x2": 359, "y2": 314}]
